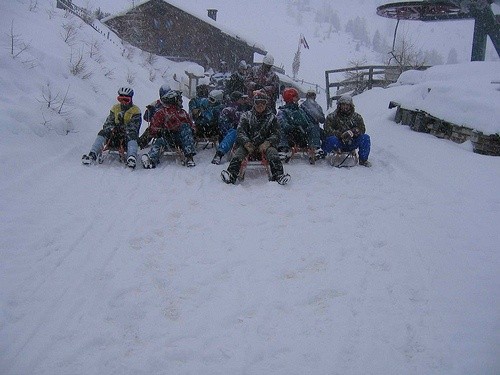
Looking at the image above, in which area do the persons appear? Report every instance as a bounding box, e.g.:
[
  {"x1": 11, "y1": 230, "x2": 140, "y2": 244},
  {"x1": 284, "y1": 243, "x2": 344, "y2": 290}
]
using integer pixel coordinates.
[
  {"x1": 143, "y1": 54, "x2": 372, "y2": 186},
  {"x1": 81, "y1": 86, "x2": 144, "y2": 171}
]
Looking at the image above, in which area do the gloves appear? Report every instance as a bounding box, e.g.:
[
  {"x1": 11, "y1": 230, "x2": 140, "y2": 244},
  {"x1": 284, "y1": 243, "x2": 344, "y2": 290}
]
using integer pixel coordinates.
[
  {"x1": 156, "y1": 126, "x2": 183, "y2": 148},
  {"x1": 329, "y1": 126, "x2": 359, "y2": 144},
  {"x1": 255, "y1": 83, "x2": 261, "y2": 89},
  {"x1": 257, "y1": 140, "x2": 270, "y2": 152},
  {"x1": 245, "y1": 141, "x2": 257, "y2": 155},
  {"x1": 113, "y1": 126, "x2": 126, "y2": 138}
]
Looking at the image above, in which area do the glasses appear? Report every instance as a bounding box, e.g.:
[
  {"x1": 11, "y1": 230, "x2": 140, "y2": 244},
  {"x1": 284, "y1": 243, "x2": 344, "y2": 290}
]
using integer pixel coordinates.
[
  {"x1": 117, "y1": 95, "x2": 131, "y2": 104},
  {"x1": 255, "y1": 94, "x2": 266, "y2": 99},
  {"x1": 208, "y1": 95, "x2": 217, "y2": 103}
]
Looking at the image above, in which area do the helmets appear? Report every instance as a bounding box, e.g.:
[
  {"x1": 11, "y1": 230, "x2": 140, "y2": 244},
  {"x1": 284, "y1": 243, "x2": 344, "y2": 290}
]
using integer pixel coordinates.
[{"x1": 117, "y1": 85, "x2": 182, "y2": 106}]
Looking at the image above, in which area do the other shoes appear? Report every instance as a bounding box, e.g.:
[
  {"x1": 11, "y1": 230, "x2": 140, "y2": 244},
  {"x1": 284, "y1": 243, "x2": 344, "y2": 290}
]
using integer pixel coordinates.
[
  {"x1": 127, "y1": 155, "x2": 137, "y2": 169},
  {"x1": 275, "y1": 146, "x2": 327, "y2": 184},
  {"x1": 359, "y1": 160, "x2": 372, "y2": 167},
  {"x1": 186, "y1": 155, "x2": 196, "y2": 166},
  {"x1": 82, "y1": 151, "x2": 97, "y2": 166},
  {"x1": 141, "y1": 153, "x2": 156, "y2": 168},
  {"x1": 212, "y1": 151, "x2": 223, "y2": 164},
  {"x1": 220, "y1": 169, "x2": 237, "y2": 184}
]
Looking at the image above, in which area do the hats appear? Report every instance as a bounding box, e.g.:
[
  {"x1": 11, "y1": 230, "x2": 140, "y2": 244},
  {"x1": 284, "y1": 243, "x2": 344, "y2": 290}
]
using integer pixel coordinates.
[
  {"x1": 283, "y1": 87, "x2": 298, "y2": 102},
  {"x1": 196, "y1": 84, "x2": 274, "y2": 105},
  {"x1": 263, "y1": 53, "x2": 274, "y2": 65},
  {"x1": 306, "y1": 91, "x2": 317, "y2": 98},
  {"x1": 238, "y1": 59, "x2": 247, "y2": 69},
  {"x1": 336, "y1": 92, "x2": 356, "y2": 109}
]
